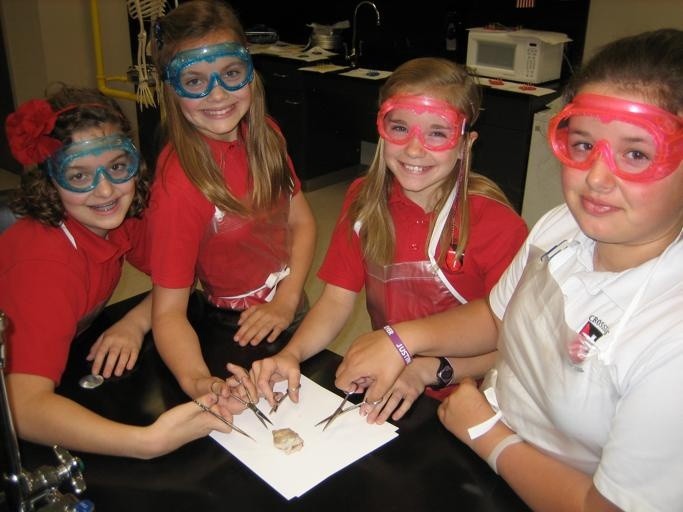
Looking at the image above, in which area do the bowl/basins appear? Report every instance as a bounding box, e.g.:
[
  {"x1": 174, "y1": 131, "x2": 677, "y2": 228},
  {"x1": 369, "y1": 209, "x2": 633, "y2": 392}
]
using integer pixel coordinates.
[{"x1": 244, "y1": 32, "x2": 278, "y2": 44}]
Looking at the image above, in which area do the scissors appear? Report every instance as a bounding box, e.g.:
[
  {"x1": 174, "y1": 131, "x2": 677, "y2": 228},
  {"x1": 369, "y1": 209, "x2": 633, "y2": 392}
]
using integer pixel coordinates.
[
  {"x1": 314, "y1": 382, "x2": 382, "y2": 431},
  {"x1": 193, "y1": 368, "x2": 301, "y2": 443}
]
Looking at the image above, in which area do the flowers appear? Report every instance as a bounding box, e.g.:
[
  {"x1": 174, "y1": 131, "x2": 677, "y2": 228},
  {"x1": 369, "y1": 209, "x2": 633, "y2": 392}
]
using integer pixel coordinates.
[{"x1": 3, "y1": 98, "x2": 63, "y2": 165}]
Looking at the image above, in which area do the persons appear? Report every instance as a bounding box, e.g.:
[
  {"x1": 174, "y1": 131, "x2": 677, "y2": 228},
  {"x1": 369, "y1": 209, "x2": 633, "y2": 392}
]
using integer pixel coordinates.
[
  {"x1": 335, "y1": 36, "x2": 683, "y2": 512},
  {"x1": 1, "y1": 87, "x2": 232, "y2": 460},
  {"x1": 145, "y1": 1, "x2": 318, "y2": 414},
  {"x1": 248, "y1": 57, "x2": 529, "y2": 425}
]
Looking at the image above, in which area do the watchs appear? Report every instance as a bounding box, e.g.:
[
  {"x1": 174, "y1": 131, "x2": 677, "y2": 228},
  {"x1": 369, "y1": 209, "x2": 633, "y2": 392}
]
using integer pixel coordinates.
[{"x1": 427, "y1": 357, "x2": 454, "y2": 391}]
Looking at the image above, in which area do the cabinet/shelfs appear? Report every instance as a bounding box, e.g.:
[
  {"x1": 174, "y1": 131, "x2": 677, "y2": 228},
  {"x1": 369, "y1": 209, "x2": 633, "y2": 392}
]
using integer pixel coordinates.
[
  {"x1": 249, "y1": 49, "x2": 356, "y2": 179},
  {"x1": 464, "y1": 83, "x2": 566, "y2": 234}
]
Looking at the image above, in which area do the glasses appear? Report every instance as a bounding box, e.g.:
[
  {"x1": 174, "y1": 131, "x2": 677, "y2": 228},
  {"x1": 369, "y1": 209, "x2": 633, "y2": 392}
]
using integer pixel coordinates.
[
  {"x1": 547, "y1": 94, "x2": 683, "y2": 182},
  {"x1": 47, "y1": 134, "x2": 142, "y2": 192},
  {"x1": 165, "y1": 39, "x2": 256, "y2": 97},
  {"x1": 378, "y1": 93, "x2": 467, "y2": 151}
]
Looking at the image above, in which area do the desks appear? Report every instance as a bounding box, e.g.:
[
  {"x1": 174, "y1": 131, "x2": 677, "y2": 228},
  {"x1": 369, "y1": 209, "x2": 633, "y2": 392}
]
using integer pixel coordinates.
[{"x1": 0, "y1": 280, "x2": 534, "y2": 510}]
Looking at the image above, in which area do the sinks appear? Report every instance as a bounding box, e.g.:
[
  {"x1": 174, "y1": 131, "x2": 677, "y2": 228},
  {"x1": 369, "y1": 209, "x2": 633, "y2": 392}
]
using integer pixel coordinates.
[{"x1": 338, "y1": 67, "x2": 394, "y2": 90}]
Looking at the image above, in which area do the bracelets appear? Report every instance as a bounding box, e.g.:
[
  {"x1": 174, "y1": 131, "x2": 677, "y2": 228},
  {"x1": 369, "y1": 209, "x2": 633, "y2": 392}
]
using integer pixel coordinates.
[
  {"x1": 486, "y1": 434, "x2": 524, "y2": 475},
  {"x1": 384, "y1": 324, "x2": 413, "y2": 366}
]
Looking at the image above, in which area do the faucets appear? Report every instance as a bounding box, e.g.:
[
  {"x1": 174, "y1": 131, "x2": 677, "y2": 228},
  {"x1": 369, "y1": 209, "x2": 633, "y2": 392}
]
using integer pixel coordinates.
[
  {"x1": 0, "y1": 312, "x2": 39, "y2": 481},
  {"x1": 342, "y1": 0, "x2": 381, "y2": 69}
]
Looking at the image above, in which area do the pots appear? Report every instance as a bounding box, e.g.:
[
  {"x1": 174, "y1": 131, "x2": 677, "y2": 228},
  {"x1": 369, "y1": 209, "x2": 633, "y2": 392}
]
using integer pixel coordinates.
[{"x1": 308, "y1": 30, "x2": 340, "y2": 50}]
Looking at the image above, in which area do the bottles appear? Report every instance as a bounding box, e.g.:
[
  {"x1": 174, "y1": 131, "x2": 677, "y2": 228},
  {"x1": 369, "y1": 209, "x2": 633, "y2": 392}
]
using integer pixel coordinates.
[
  {"x1": 515, "y1": 1, "x2": 534, "y2": 9},
  {"x1": 446, "y1": 19, "x2": 457, "y2": 52}
]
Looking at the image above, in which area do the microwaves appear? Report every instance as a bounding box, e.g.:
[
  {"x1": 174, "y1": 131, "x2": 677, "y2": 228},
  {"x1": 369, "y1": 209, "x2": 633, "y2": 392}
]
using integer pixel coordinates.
[{"x1": 462, "y1": 31, "x2": 565, "y2": 83}]
